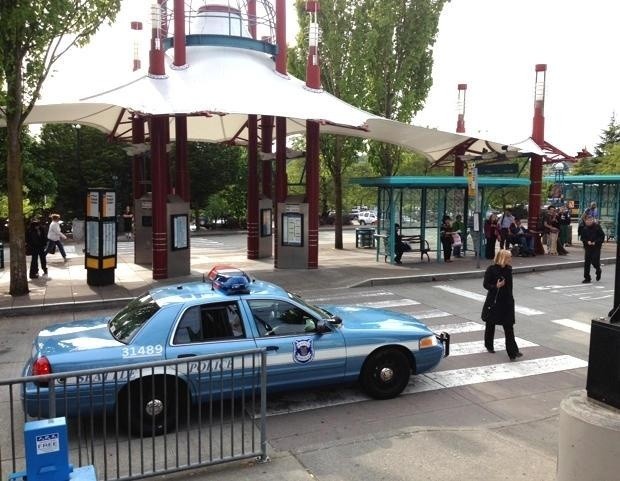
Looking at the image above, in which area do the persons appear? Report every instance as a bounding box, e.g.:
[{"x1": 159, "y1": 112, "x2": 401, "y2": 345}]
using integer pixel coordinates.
[
  {"x1": 580, "y1": 215, "x2": 606, "y2": 283},
  {"x1": 26, "y1": 222, "x2": 48, "y2": 278},
  {"x1": 122, "y1": 206, "x2": 134, "y2": 240},
  {"x1": 386, "y1": 223, "x2": 405, "y2": 264},
  {"x1": 485, "y1": 200, "x2": 599, "y2": 259},
  {"x1": 44, "y1": 213, "x2": 69, "y2": 265},
  {"x1": 440, "y1": 215, "x2": 456, "y2": 264},
  {"x1": 481, "y1": 250, "x2": 524, "y2": 360},
  {"x1": 452, "y1": 215, "x2": 466, "y2": 258}
]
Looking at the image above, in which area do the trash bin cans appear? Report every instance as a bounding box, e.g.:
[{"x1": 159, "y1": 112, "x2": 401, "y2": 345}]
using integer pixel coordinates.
[{"x1": 355, "y1": 228, "x2": 375, "y2": 248}]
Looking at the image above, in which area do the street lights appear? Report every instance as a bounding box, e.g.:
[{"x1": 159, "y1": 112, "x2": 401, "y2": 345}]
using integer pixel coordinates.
[
  {"x1": 70, "y1": 121, "x2": 84, "y2": 220},
  {"x1": 552, "y1": 161, "x2": 566, "y2": 206}
]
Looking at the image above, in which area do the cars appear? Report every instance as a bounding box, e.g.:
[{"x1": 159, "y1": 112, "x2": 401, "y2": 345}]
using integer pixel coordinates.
[
  {"x1": 15, "y1": 263, "x2": 457, "y2": 442},
  {"x1": 45, "y1": 220, "x2": 72, "y2": 233},
  {"x1": 329, "y1": 203, "x2": 498, "y2": 226}
]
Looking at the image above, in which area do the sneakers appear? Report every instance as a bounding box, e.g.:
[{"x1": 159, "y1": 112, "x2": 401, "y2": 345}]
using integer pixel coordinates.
[
  {"x1": 444, "y1": 260, "x2": 452, "y2": 263},
  {"x1": 596, "y1": 269, "x2": 601, "y2": 281},
  {"x1": 582, "y1": 279, "x2": 591, "y2": 283},
  {"x1": 44, "y1": 267, "x2": 47, "y2": 273},
  {"x1": 454, "y1": 256, "x2": 462, "y2": 258},
  {"x1": 30, "y1": 275, "x2": 38, "y2": 278},
  {"x1": 510, "y1": 353, "x2": 522, "y2": 361},
  {"x1": 486, "y1": 346, "x2": 495, "y2": 353},
  {"x1": 394, "y1": 258, "x2": 401, "y2": 264},
  {"x1": 64, "y1": 258, "x2": 71, "y2": 262}
]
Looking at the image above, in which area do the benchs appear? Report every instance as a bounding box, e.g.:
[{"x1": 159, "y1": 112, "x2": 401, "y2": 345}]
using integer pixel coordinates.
[{"x1": 383, "y1": 233, "x2": 431, "y2": 263}]
